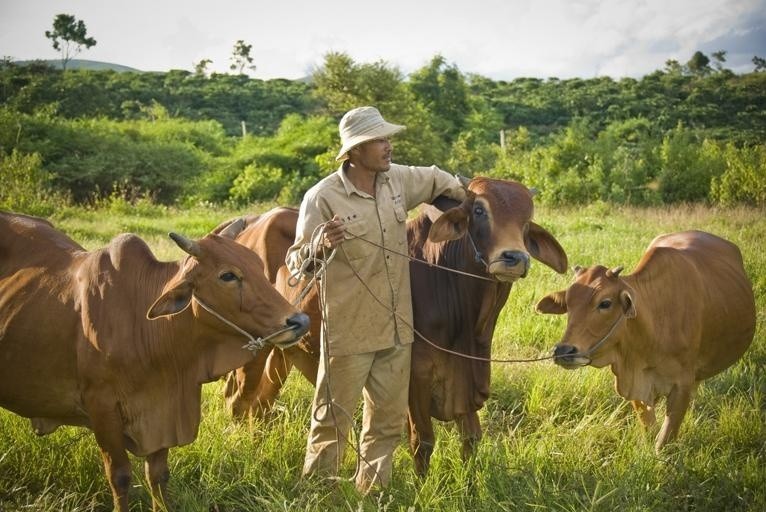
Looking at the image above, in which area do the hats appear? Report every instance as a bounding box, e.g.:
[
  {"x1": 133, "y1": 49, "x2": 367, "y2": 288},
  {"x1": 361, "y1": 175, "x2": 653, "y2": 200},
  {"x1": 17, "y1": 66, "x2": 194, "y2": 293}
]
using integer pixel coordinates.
[{"x1": 335, "y1": 104, "x2": 409, "y2": 164}]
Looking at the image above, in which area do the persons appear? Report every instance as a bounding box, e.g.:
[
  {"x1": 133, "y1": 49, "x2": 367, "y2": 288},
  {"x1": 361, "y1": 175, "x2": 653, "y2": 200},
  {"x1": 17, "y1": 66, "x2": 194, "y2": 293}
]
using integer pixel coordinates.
[{"x1": 284, "y1": 107, "x2": 468, "y2": 491}]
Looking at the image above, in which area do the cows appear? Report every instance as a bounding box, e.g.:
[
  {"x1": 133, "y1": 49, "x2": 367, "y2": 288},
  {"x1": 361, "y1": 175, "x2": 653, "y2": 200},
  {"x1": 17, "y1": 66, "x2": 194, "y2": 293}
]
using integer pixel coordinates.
[
  {"x1": 536, "y1": 230, "x2": 757, "y2": 459},
  {"x1": 0, "y1": 208, "x2": 312, "y2": 512},
  {"x1": 211, "y1": 177, "x2": 568, "y2": 512}
]
